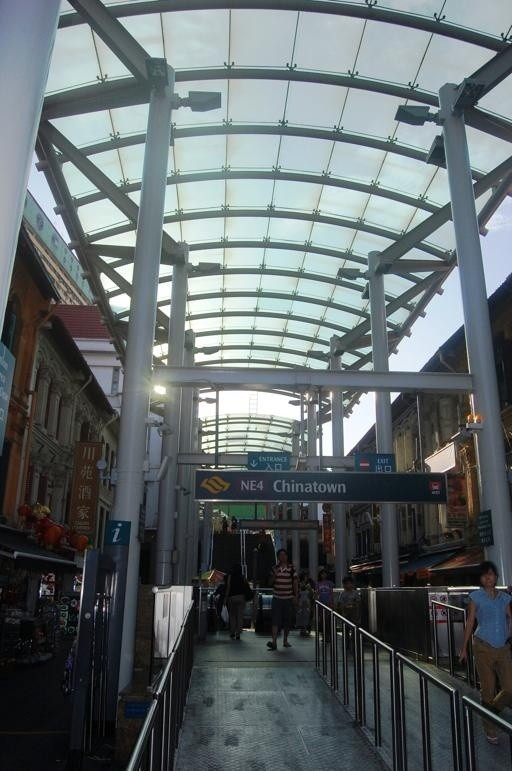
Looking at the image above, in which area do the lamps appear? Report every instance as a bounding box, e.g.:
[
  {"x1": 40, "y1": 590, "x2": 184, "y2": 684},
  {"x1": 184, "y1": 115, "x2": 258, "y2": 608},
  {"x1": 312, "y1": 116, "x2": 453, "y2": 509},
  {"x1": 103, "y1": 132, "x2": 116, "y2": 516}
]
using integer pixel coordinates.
[
  {"x1": 193, "y1": 345, "x2": 222, "y2": 357},
  {"x1": 288, "y1": 398, "x2": 315, "y2": 406},
  {"x1": 186, "y1": 256, "x2": 220, "y2": 274},
  {"x1": 305, "y1": 350, "x2": 333, "y2": 364},
  {"x1": 169, "y1": 87, "x2": 222, "y2": 115},
  {"x1": 335, "y1": 267, "x2": 371, "y2": 283},
  {"x1": 145, "y1": 416, "x2": 173, "y2": 439},
  {"x1": 199, "y1": 429, "x2": 218, "y2": 437},
  {"x1": 276, "y1": 431, "x2": 295, "y2": 439},
  {"x1": 464, "y1": 413, "x2": 485, "y2": 432},
  {"x1": 197, "y1": 395, "x2": 217, "y2": 405},
  {"x1": 392, "y1": 104, "x2": 442, "y2": 127}
]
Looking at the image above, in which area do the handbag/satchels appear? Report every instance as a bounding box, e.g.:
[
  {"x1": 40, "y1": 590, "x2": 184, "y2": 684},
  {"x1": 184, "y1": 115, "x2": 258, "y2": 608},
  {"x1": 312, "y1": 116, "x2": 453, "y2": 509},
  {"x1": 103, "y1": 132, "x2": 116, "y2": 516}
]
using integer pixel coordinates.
[{"x1": 226, "y1": 594, "x2": 246, "y2": 608}]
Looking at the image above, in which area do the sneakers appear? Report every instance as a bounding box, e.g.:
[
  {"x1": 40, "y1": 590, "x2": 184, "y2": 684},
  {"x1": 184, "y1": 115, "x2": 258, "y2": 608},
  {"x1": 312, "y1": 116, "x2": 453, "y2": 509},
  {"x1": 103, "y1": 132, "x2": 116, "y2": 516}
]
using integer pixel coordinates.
[
  {"x1": 283, "y1": 642, "x2": 292, "y2": 647},
  {"x1": 229, "y1": 632, "x2": 241, "y2": 642},
  {"x1": 267, "y1": 641, "x2": 277, "y2": 650}
]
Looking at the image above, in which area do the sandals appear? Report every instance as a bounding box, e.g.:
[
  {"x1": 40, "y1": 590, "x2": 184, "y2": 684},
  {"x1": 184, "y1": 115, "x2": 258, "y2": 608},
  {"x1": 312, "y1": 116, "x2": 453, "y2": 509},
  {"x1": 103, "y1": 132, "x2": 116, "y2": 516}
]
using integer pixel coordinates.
[{"x1": 484, "y1": 731, "x2": 499, "y2": 745}]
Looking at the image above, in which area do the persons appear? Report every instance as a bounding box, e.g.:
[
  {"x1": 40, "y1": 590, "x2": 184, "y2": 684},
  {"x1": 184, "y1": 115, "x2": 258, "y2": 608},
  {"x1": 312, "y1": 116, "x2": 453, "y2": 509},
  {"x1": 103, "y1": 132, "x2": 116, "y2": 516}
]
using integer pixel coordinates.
[
  {"x1": 404, "y1": 572, "x2": 408, "y2": 588},
  {"x1": 460, "y1": 560, "x2": 511, "y2": 745},
  {"x1": 410, "y1": 571, "x2": 418, "y2": 586},
  {"x1": 211, "y1": 548, "x2": 360, "y2": 649}
]
